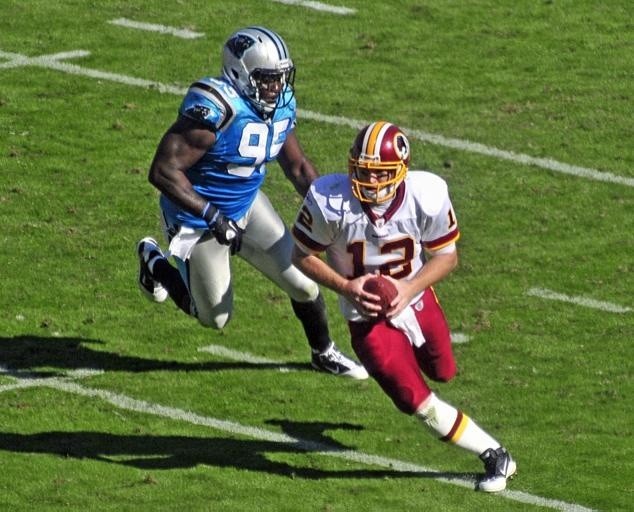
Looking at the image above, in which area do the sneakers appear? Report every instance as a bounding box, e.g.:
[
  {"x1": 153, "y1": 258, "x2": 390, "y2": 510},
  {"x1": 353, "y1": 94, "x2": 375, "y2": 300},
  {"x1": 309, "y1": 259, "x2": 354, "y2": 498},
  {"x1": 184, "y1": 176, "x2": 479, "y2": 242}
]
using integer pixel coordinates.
[
  {"x1": 137, "y1": 237, "x2": 168, "y2": 302},
  {"x1": 309, "y1": 342, "x2": 369, "y2": 379},
  {"x1": 480, "y1": 442, "x2": 517, "y2": 493}
]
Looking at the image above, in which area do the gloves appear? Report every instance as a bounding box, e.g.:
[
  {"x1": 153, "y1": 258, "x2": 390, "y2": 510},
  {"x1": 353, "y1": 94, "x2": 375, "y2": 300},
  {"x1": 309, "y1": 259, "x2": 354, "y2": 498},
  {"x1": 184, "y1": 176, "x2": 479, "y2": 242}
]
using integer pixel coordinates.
[{"x1": 210, "y1": 214, "x2": 241, "y2": 247}]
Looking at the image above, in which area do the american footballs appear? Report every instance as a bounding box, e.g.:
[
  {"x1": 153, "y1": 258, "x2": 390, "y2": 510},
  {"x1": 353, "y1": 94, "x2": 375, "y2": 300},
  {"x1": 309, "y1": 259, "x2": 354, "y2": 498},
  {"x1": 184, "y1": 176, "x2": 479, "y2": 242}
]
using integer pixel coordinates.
[{"x1": 363, "y1": 277, "x2": 398, "y2": 320}]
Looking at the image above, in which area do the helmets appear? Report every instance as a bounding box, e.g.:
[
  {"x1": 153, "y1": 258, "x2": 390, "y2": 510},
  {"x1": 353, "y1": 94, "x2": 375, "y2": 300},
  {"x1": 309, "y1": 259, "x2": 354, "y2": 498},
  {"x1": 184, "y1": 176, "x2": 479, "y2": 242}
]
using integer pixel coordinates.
[
  {"x1": 348, "y1": 121, "x2": 411, "y2": 206},
  {"x1": 222, "y1": 28, "x2": 296, "y2": 112}
]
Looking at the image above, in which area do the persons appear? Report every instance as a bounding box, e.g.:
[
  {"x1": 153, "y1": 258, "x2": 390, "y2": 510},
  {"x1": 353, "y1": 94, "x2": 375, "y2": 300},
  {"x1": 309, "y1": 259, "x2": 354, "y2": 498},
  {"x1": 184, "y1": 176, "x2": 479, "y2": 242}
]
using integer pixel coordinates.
[
  {"x1": 136, "y1": 22, "x2": 370, "y2": 379},
  {"x1": 289, "y1": 121, "x2": 518, "y2": 492}
]
